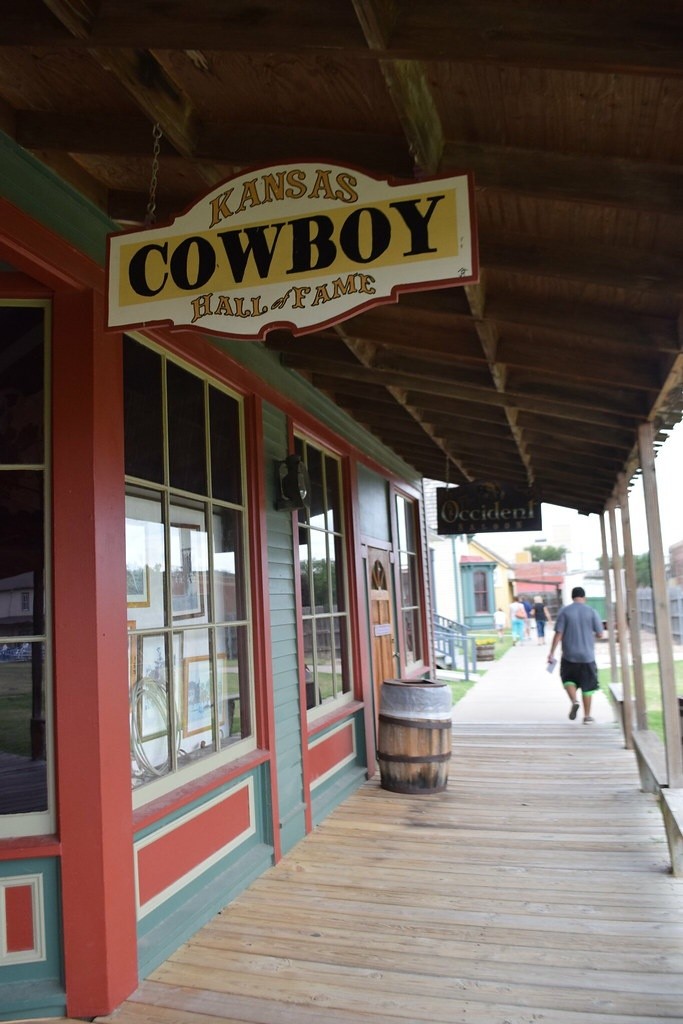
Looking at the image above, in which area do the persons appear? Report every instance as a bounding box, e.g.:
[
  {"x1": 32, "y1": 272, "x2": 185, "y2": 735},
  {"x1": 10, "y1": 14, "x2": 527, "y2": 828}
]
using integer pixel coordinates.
[
  {"x1": 529, "y1": 597, "x2": 552, "y2": 645},
  {"x1": 548, "y1": 587, "x2": 604, "y2": 723},
  {"x1": 509, "y1": 596, "x2": 531, "y2": 647},
  {"x1": 494, "y1": 608, "x2": 506, "y2": 641}
]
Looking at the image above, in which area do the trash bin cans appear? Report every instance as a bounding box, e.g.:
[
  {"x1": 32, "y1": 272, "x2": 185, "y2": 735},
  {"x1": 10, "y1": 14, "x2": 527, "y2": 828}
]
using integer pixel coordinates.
[{"x1": 377, "y1": 678, "x2": 453, "y2": 794}]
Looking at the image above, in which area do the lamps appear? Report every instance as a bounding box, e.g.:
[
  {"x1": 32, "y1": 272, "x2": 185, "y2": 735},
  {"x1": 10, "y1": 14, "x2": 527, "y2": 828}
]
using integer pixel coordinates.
[{"x1": 275, "y1": 453, "x2": 309, "y2": 512}]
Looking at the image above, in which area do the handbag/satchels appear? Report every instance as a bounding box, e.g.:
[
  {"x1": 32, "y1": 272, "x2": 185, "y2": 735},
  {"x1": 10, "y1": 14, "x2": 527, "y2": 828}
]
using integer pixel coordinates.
[{"x1": 514, "y1": 607, "x2": 528, "y2": 620}]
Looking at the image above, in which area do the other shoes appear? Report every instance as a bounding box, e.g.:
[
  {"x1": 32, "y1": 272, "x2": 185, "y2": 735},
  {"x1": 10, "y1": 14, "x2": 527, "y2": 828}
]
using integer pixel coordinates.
[
  {"x1": 583, "y1": 717, "x2": 595, "y2": 726},
  {"x1": 568, "y1": 701, "x2": 580, "y2": 720}
]
